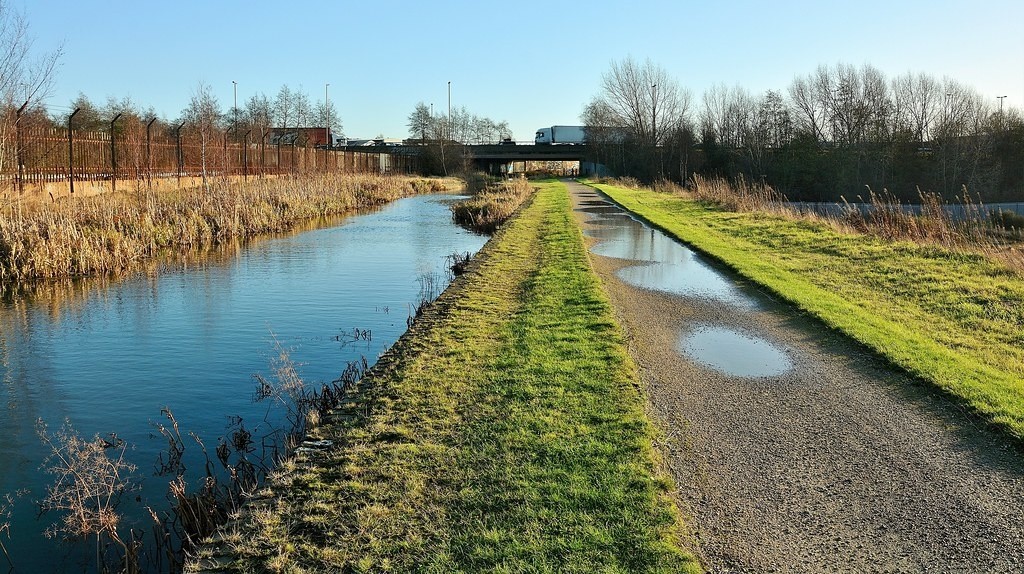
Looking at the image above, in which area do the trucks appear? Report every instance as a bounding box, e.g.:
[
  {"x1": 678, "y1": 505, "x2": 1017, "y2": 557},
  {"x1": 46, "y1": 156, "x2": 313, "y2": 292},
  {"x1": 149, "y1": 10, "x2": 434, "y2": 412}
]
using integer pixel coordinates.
[{"x1": 535, "y1": 125, "x2": 636, "y2": 146}]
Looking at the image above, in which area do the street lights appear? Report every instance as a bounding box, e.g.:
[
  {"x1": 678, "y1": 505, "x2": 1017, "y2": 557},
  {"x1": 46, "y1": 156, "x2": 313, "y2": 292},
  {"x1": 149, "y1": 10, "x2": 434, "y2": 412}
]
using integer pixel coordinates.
[{"x1": 997, "y1": 95, "x2": 1008, "y2": 125}]
